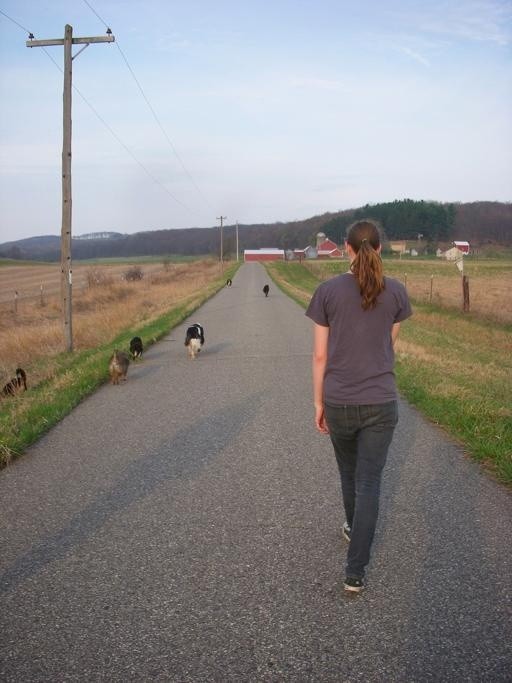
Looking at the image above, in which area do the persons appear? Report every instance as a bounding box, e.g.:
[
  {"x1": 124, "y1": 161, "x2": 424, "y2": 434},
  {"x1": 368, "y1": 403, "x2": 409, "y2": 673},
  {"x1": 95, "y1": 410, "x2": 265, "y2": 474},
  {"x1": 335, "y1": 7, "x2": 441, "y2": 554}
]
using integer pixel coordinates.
[{"x1": 304, "y1": 219, "x2": 414, "y2": 592}]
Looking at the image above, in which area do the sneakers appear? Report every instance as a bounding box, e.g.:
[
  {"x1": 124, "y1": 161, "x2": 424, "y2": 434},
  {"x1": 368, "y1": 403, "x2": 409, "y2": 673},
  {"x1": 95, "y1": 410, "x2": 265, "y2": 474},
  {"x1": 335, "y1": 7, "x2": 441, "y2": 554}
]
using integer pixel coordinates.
[
  {"x1": 342, "y1": 521, "x2": 351, "y2": 542},
  {"x1": 343, "y1": 577, "x2": 365, "y2": 592}
]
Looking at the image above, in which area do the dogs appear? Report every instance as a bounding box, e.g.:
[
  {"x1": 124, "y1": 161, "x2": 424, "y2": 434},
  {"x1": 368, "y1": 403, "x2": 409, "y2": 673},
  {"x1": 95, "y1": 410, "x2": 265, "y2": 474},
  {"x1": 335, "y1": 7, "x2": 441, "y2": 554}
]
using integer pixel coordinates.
[
  {"x1": 184, "y1": 324, "x2": 204, "y2": 360},
  {"x1": 0, "y1": 368, "x2": 27, "y2": 398},
  {"x1": 263, "y1": 285, "x2": 269, "y2": 296},
  {"x1": 108, "y1": 349, "x2": 129, "y2": 385},
  {"x1": 227, "y1": 279, "x2": 232, "y2": 289},
  {"x1": 129, "y1": 337, "x2": 143, "y2": 360}
]
